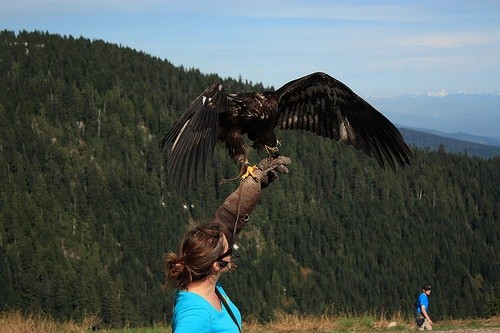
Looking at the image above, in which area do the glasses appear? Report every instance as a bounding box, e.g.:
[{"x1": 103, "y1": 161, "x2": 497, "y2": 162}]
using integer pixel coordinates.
[{"x1": 214, "y1": 245, "x2": 232, "y2": 262}]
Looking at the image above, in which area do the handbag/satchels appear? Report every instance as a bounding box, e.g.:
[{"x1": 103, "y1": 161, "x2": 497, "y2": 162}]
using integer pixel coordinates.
[{"x1": 416, "y1": 314, "x2": 425, "y2": 326}]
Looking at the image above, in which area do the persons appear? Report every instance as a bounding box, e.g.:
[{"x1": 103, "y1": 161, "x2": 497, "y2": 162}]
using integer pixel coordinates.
[
  {"x1": 165, "y1": 155, "x2": 292, "y2": 333},
  {"x1": 416, "y1": 284, "x2": 434, "y2": 333}
]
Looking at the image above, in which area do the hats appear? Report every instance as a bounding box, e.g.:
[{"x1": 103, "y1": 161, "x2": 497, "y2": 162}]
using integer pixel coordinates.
[{"x1": 423, "y1": 284, "x2": 432, "y2": 290}]
